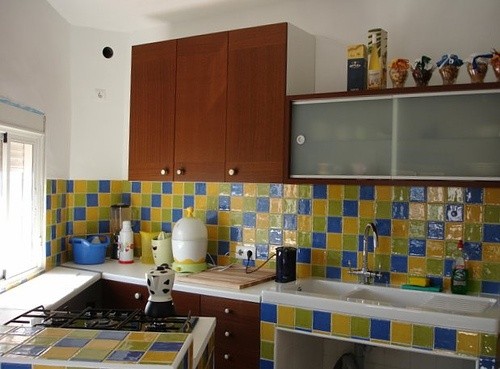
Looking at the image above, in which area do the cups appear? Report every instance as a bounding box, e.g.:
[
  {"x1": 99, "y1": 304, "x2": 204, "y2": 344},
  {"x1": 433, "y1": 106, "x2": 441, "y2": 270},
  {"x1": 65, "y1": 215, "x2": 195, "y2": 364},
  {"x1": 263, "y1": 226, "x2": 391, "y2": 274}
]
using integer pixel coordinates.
[{"x1": 151, "y1": 236, "x2": 174, "y2": 269}]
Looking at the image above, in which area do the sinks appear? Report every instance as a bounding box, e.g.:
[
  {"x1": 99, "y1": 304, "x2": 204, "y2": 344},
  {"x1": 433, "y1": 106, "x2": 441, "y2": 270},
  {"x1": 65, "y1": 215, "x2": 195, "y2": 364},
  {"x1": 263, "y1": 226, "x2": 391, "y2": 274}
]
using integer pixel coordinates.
[
  {"x1": 262, "y1": 278, "x2": 359, "y2": 316},
  {"x1": 343, "y1": 286, "x2": 500, "y2": 334}
]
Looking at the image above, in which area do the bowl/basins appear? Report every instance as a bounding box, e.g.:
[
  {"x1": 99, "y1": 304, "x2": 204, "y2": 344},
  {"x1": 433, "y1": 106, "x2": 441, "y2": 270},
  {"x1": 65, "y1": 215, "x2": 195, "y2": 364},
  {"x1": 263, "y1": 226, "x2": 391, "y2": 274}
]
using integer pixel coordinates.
[{"x1": 69, "y1": 235, "x2": 110, "y2": 265}]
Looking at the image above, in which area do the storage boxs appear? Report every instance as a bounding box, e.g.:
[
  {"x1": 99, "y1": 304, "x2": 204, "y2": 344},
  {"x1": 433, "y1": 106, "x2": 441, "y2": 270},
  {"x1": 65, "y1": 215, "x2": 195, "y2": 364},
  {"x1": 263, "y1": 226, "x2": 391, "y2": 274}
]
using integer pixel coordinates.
[
  {"x1": 366, "y1": 27, "x2": 387, "y2": 90},
  {"x1": 347, "y1": 44, "x2": 367, "y2": 92}
]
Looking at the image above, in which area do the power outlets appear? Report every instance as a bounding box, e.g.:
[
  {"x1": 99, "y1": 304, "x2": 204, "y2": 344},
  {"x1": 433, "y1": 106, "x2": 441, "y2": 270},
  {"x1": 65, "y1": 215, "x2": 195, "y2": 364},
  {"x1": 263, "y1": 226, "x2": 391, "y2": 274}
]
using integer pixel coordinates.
[
  {"x1": 96, "y1": 90, "x2": 105, "y2": 101},
  {"x1": 235, "y1": 246, "x2": 256, "y2": 261}
]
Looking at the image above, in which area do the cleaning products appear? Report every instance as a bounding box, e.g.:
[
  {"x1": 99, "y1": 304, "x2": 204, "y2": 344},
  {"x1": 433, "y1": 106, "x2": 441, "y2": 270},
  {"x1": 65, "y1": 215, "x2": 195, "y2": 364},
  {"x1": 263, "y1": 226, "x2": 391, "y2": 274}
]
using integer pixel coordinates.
[{"x1": 450, "y1": 239, "x2": 469, "y2": 293}]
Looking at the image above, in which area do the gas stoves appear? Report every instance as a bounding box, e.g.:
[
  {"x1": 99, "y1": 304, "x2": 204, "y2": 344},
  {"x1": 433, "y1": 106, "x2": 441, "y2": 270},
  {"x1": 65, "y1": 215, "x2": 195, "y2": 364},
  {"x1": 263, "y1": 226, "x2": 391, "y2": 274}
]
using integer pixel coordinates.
[{"x1": 2, "y1": 305, "x2": 198, "y2": 333}]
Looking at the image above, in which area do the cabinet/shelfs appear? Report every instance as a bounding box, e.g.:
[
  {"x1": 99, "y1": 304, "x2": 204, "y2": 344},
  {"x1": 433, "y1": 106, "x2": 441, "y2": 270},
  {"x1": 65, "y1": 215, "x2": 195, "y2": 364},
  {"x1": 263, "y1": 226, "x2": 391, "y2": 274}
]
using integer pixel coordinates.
[
  {"x1": 56, "y1": 280, "x2": 259, "y2": 369},
  {"x1": 284, "y1": 82, "x2": 500, "y2": 188},
  {"x1": 128, "y1": 32, "x2": 228, "y2": 183},
  {"x1": 225, "y1": 22, "x2": 315, "y2": 183}
]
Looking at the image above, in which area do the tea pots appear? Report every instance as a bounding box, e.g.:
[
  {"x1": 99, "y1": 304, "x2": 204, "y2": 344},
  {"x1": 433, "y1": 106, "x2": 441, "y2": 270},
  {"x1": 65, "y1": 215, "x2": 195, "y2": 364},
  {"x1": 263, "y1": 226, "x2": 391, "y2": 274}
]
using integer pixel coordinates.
[{"x1": 145, "y1": 263, "x2": 175, "y2": 302}]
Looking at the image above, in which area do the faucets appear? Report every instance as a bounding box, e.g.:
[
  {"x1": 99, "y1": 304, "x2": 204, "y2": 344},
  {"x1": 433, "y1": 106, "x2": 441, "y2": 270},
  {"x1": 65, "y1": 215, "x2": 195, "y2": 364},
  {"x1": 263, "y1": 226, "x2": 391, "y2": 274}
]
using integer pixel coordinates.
[{"x1": 347, "y1": 222, "x2": 379, "y2": 284}]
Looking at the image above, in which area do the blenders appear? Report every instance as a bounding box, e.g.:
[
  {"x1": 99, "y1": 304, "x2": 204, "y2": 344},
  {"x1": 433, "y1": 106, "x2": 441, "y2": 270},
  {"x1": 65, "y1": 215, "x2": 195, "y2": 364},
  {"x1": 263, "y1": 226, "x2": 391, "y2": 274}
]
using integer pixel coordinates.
[{"x1": 110, "y1": 204, "x2": 131, "y2": 260}]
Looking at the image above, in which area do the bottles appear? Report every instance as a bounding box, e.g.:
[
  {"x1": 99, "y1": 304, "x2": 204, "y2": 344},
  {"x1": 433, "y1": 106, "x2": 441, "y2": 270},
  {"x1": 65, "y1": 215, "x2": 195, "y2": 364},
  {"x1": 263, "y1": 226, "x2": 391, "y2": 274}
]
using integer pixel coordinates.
[
  {"x1": 450, "y1": 240, "x2": 469, "y2": 295},
  {"x1": 439, "y1": 55, "x2": 458, "y2": 85},
  {"x1": 118, "y1": 221, "x2": 134, "y2": 264},
  {"x1": 491, "y1": 53, "x2": 500, "y2": 82},
  {"x1": 467, "y1": 58, "x2": 488, "y2": 83},
  {"x1": 389, "y1": 59, "x2": 408, "y2": 88},
  {"x1": 368, "y1": 34, "x2": 380, "y2": 89},
  {"x1": 412, "y1": 62, "x2": 433, "y2": 87}
]
